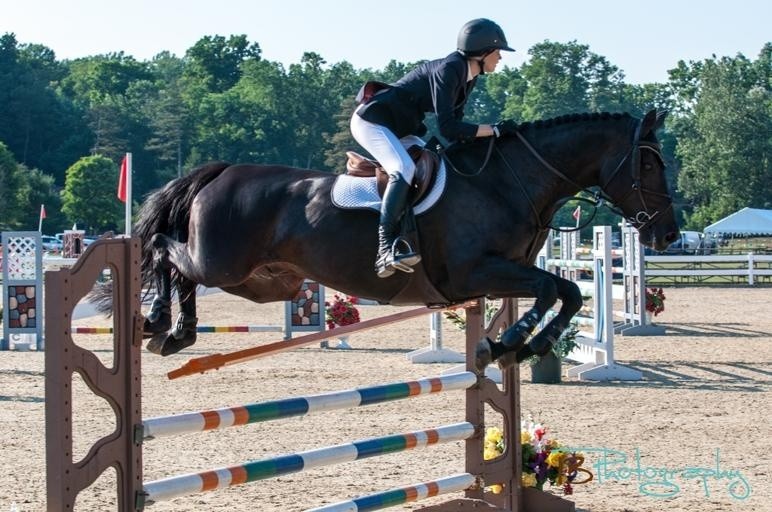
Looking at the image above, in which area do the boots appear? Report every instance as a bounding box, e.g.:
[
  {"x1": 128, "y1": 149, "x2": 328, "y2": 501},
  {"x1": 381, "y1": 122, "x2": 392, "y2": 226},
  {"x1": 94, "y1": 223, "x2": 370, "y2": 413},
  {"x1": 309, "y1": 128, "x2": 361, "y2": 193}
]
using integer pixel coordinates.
[{"x1": 375, "y1": 170, "x2": 422, "y2": 278}]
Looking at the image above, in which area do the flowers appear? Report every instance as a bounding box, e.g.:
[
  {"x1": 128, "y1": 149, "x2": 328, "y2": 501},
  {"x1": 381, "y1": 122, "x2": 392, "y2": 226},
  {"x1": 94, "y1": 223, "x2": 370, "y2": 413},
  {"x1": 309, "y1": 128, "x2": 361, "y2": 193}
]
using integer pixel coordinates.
[
  {"x1": 479, "y1": 421, "x2": 592, "y2": 494},
  {"x1": 323, "y1": 294, "x2": 362, "y2": 330},
  {"x1": 522, "y1": 311, "x2": 580, "y2": 362},
  {"x1": 645, "y1": 289, "x2": 669, "y2": 315}
]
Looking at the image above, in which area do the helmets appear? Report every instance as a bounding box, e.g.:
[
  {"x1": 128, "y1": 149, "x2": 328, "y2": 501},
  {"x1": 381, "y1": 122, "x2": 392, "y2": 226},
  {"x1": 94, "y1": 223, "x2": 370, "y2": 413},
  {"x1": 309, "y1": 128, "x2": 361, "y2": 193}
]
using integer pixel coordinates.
[{"x1": 457, "y1": 17, "x2": 515, "y2": 52}]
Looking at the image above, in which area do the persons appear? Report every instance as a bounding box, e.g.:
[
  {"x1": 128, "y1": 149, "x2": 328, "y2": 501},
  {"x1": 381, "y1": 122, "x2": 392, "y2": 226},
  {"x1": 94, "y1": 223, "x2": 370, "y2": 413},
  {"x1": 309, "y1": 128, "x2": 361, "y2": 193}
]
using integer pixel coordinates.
[{"x1": 349, "y1": 19, "x2": 503, "y2": 277}]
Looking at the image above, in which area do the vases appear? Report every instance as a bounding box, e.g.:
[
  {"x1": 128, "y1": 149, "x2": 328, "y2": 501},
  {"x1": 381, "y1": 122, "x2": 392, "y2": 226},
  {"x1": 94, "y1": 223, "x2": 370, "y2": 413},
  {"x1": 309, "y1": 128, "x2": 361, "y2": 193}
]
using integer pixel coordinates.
[
  {"x1": 335, "y1": 330, "x2": 352, "y2": 349},
  {"x1": 530, "y1": 355, "x2": 563, "y2": 385},
  {"x1": 645, "y1": 308, "x2": 655, "y2": 327}
]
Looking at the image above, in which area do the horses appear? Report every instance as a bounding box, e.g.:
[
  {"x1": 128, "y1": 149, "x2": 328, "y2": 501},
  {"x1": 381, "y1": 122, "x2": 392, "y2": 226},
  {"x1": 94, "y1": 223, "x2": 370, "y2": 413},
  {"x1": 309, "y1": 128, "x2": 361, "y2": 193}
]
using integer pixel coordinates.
[{"x1": 85, "y1": 108, "x2": 679, "y2": 372}]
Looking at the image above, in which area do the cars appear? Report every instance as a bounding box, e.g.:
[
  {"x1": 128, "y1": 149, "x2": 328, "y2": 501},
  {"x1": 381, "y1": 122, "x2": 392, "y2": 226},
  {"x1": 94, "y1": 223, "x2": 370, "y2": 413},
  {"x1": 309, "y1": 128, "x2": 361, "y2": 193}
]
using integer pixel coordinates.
[
  {"x1": 609, "y1": 230, "x2": 718, "y2": 256},
  {"x1": 41, "y1": 233, "x2": 94, "y2": 254}
]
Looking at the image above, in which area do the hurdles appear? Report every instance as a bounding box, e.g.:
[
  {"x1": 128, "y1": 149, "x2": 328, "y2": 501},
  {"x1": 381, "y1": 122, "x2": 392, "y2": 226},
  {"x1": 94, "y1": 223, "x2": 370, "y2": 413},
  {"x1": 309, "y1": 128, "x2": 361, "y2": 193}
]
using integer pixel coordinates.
[
  {"x1": 45, "y1": 239, "x2": 522, "y2": 512},
  {"x1": 536, "y1": 226, "x2": 645, "y2": 382},
  {"x1": 411, "y1": 312, "x2": 467, "y2": 363},
  {"x1": 543, "y1": 226, "x2": 667, "y2": 337},
  {"x1": 0, "y1": 231, "x2": 329, "y2": 352}
]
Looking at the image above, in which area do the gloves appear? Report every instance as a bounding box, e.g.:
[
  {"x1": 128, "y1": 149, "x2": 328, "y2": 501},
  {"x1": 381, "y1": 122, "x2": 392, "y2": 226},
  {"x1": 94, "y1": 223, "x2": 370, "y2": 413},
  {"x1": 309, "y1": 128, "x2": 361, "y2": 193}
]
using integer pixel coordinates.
[{"x1": 492, "y1": 119, "x2": 517, "y2": 139}]
